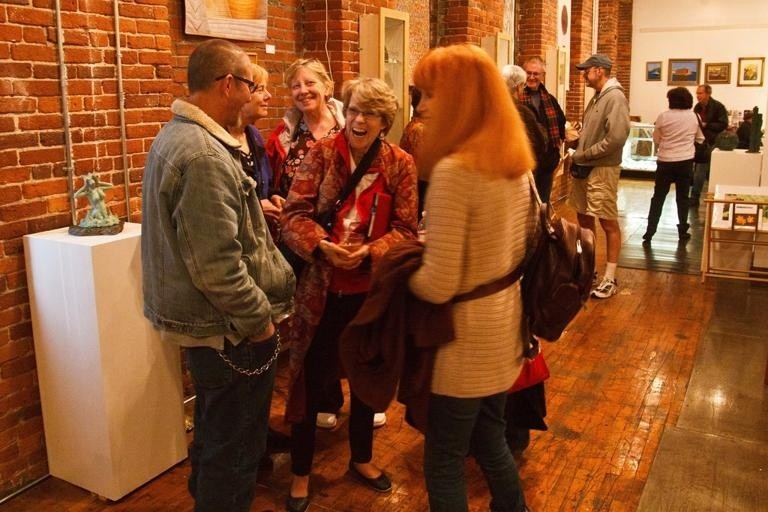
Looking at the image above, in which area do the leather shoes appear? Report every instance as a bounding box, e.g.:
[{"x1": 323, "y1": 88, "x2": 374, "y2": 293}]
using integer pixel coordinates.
[
  {"x1": 287, "y1": 476, "x2": 312, "y2": 512},
  {"x1": 348, "y1": 458, "x2": 393, "y2": 493}
]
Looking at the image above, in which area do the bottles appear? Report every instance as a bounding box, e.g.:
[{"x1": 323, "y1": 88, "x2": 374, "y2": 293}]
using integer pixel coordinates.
[{"x1": 417, "y1": 210, "x2": 427, "y2": 242}]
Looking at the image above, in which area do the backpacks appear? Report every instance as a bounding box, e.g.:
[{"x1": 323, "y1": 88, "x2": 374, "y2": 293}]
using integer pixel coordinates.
[{"x1": 453, "y1": 169, "x2": 596, "y2": 342}]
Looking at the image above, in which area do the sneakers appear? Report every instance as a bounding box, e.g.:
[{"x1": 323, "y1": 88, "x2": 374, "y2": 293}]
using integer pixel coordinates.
[
  {"x1": 373, "y1": 412, "x2": 386, "y2": 428},
  {"x1": 590, "y1": 275, "x2": 618, "y2": 299},
  {"x1": 316, "y1": 412, "x2": 337, "y2": 428}
]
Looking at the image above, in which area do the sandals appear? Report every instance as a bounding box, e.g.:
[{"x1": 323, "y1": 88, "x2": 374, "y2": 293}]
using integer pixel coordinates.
[{"x1": 642, "y1": 226, "x2": 656, "y2": 241}]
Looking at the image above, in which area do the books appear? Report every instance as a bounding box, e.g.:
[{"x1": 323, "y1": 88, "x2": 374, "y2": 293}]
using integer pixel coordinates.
[{"x1": 712, "y1": 184, "x2": 767, "y2": 234}]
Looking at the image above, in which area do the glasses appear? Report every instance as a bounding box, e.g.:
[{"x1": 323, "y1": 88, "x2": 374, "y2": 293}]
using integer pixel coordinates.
[
  {"x1": 343, "y1": 105, "x2": 383, "y2": 122},
  {"x1": 214, "y1": 73, "x2": 259, "y2": 95}
]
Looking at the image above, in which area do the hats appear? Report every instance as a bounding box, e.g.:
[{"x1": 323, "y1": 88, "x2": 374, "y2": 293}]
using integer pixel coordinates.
[{"x1": 574, "y1": 54, "x2": 612, "y2": 69}]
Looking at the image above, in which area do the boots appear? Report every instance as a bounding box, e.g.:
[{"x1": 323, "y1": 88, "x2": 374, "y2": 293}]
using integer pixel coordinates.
[{"x1": 676, "y1": 224, "x2": 690, "y2": 240}]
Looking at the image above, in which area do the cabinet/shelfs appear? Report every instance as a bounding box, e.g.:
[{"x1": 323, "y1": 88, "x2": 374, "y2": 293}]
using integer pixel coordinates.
[
  {"x1": 700, "y1": 192, "x2": 768, "y2": 284},
  {"x1": 622, "y1": 124, "x2": 658, "y2": 172}
]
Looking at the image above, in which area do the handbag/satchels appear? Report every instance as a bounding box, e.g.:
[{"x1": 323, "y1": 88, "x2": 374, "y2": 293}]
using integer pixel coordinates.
[
  {"x1": 695, "y1": 141, "x2": 709, "y2": 165},
  {"x1": 570, "y1": 162, "x2": 593, "y2": 178}
]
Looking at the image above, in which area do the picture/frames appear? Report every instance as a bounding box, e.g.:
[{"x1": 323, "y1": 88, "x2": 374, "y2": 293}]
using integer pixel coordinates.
[
  {"x1": 646, "y1": 61, "x2": 662, "y2": 81},
  {"x1": 668, "y1": 58, "x2": 701, "y2": 86},
  {"x1": 706, "y1": 63, "x2": 731, "y2": 84},
  {"x1": 737, "y1": 57, "x2": 765, "y2": 86}
]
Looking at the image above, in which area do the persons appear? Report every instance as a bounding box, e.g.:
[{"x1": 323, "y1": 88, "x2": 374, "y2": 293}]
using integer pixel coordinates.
[
  {"x1": 139, "y1": 36, "x2": 308, "y2": 512},
  {"x1": 75, "y1": 170, "x2": 121, "y2": 228},
  {"x1": 517, "y1": 54, "x2": 569, "y2": 206},
  {"x1": 395, "y1": 82, "x2": 433, "y2": 217},
  {"x1": 641, "y1": 85, "x2": 708, "y2": 244},
  {"x1": 258, "y1": 53, "x2": 391, "y2": 436},
  {"x1": 570, "y1": 53, "x2": 632, "y2": 304},
  {"x1": 277, "y1": 76, "x2": 425, "y2": 512},
  {"x1": 499, "y1": 62, "x2": 550, "y2": 198},
  {"x1": 400, "y1": 40, "x2": 550, "y2": 511},
  {"x1": 689, "y1": 84, "x2": 730, "y2": 208},
  {"x1": 229, "y1": 61, "x2": 298, "y2": 475},
  {"x1": 735, "y1": 110, "x2": 753, "y2": 148}
]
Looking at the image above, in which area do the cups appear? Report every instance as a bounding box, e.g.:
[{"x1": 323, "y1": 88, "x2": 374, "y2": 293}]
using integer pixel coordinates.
[
  {"x1": 338, "y1": 232, "x2": 365, "y2": 260},
  {"x1": 713, "y1": 185, "x2": 726, "y2": 201}
]
